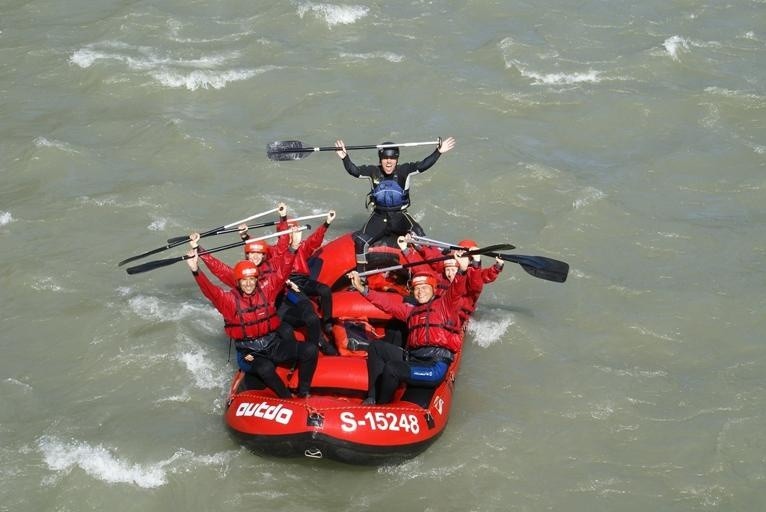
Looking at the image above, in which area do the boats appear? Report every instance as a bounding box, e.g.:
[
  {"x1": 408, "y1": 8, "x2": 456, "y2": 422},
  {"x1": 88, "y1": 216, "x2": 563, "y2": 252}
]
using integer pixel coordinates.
[{"x1": 222, "y1": 230, "x2": 468, "y2": 455}]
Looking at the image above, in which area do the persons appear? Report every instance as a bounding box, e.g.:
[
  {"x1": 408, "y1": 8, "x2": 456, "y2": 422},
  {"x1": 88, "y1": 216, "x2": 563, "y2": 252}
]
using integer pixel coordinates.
[{"x1": 334, "y1": 134, "x2": 457, "y2": 292}]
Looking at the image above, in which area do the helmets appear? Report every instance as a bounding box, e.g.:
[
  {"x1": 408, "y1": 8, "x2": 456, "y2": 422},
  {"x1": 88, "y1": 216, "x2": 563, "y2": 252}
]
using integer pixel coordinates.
[
  {"x1": 412, "y1": 271, "x2": 437, "y2": 290},
  {"x1": 244, "y1": 239, "x2": 267, "y2": 253},
  {"x1": 441, "y1": 259, "x2": 460, "y2": 268},
  {"x1": 379, "y1": 142, "x2": 399, "y2": 158},
  {"x1": 233, "y1": 260, "x2": 259, "y2": 281}
]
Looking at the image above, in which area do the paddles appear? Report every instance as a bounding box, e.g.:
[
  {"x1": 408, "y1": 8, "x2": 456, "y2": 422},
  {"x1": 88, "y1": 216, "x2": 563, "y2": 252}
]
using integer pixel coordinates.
[
  {"x1": 343, "y1": 244, "x2": 515, "y2": 279},
  {"x1": 267, "y1": 137, "x2": 442, "y2": 161},
  {"x1": 118, "y1": 208, "x2": 335, "y2": 275},
  {"x1": 408, "y1": 235, "x2": 569, "y2": 282}
]
